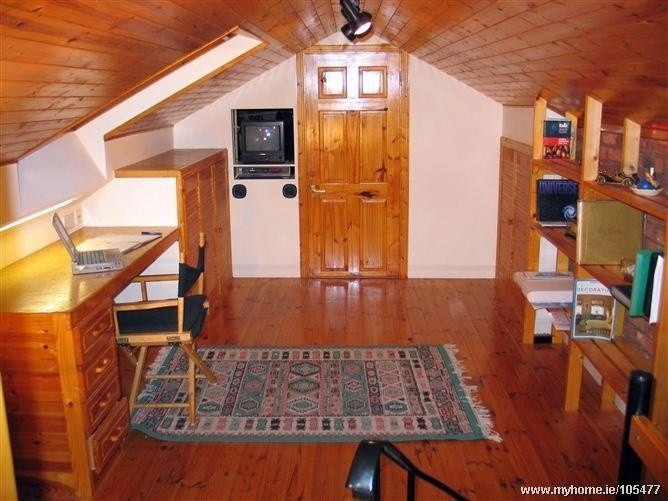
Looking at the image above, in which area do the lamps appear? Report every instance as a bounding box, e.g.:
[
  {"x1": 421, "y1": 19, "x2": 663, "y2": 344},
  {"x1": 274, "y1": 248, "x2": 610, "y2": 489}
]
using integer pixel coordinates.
[{"x1": 340, "y1": 0, "x2": 372, "y2": 42}]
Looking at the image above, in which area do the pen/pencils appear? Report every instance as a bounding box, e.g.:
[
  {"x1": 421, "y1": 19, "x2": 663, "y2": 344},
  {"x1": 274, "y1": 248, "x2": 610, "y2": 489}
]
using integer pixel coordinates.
[{"x1": 142, "y1": 232, "x2": 161, "y2": 235}]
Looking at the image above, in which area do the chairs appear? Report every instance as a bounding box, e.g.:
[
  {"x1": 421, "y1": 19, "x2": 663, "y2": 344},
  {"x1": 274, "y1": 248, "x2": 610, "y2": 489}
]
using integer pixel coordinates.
[{"x1": 114, "y1": 232, "x2": 218, "y2": 423}]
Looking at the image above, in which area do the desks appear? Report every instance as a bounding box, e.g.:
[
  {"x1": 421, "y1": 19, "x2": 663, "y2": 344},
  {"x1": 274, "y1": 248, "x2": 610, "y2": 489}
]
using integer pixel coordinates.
[{"x1": 0, "y1": 226, "x2": 181, "y2": 501}]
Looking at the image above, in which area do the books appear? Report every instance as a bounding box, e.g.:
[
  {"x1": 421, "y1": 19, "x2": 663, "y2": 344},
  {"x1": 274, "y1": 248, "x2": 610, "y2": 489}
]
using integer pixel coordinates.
[
  {"x1": 535, "y1": 178, "x2": 578, "y2": 223},
  {"x1": 513, "y1": 247, "x2": 664, "y2": 340},
  {"x1": 542, "y1": 118, "x2": 572, "y2": 161},
  {"x1": 76, "y1": 233, "x2": 161, "y2": 255}
]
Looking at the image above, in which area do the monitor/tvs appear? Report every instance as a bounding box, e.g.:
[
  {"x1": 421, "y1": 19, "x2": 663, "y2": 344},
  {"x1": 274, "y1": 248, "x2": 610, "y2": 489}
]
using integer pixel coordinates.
[{"x1": 240, "y1": 121, "x2": 285, "y2": 163}]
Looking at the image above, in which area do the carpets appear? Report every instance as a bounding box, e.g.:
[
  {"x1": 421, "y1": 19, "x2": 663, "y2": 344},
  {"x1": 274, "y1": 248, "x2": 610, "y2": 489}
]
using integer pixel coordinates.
[{"x1": 131, "y1": 343, "x2": 502, "y2": 444}]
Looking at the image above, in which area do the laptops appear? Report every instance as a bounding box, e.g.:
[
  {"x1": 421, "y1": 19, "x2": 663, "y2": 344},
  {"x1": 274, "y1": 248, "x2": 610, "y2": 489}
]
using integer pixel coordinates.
[{"x1": 51, "y1": 206, "x2": 125, "y2": 274}]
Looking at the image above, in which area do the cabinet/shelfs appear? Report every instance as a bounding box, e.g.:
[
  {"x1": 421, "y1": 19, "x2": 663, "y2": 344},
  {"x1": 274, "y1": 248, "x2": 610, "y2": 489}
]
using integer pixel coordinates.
[{"x1": 522, "y1": 92, "x2": 668, "y2": 494}]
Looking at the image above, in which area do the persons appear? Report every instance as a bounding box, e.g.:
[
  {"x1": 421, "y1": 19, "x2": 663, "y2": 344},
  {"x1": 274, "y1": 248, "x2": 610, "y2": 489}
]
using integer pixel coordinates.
[{"x1": 596, "y1": 169, "x2": 634, "y2": 188}]
